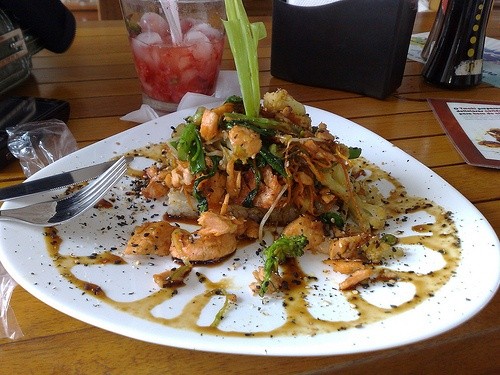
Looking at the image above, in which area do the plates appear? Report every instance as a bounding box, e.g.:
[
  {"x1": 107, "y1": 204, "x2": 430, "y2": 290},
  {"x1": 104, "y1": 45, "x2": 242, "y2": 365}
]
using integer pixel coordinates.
[{"x1": 0, "y1": 96, "x2": 500, "y2": 356}]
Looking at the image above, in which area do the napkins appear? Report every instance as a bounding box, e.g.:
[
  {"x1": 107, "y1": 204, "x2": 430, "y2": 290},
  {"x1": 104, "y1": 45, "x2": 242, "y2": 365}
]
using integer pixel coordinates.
[{"x1": 120, "y1": 70, "x2": 242, "y2": 123}]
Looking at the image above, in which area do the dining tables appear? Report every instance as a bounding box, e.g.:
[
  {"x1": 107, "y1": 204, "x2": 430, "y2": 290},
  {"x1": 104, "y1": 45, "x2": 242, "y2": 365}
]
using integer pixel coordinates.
[{"x1": 0, "y1": 0, "x2": 500, "y2": 375}]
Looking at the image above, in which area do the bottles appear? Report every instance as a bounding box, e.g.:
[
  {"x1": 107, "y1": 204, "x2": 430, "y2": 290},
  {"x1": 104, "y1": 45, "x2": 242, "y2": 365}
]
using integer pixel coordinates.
[{"x1": 418, "y1": 0, "x2": 495, "y2": 93}]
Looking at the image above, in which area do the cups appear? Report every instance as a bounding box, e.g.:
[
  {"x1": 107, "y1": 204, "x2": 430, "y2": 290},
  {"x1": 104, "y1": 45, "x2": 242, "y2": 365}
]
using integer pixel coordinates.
[{"x1": 116, "y1": 0, "x2": 227, "y2": 112}]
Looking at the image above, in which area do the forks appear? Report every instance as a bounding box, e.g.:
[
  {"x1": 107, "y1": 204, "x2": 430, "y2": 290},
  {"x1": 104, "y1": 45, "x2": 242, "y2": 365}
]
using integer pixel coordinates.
[{"x1": 0, "y1": 156, "x2": 128, "y2": 227}]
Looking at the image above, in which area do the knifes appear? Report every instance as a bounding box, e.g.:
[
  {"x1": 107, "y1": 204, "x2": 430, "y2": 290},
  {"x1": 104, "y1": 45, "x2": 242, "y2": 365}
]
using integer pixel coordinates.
[{"x1": 0, "y1": 156, "x2": 133, "y2": 200}]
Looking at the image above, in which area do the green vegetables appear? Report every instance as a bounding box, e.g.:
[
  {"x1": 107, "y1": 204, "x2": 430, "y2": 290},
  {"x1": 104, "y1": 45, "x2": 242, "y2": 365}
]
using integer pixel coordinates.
[{"x1": 170, "y1": 0, "x2": 362, "y2": 297}]
